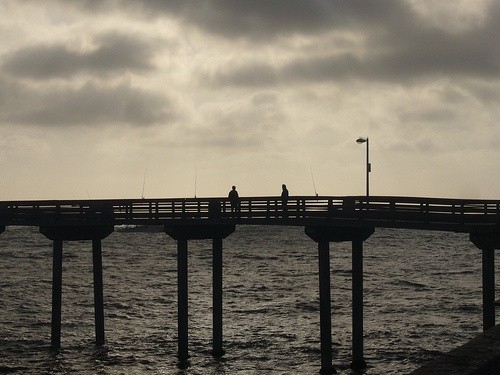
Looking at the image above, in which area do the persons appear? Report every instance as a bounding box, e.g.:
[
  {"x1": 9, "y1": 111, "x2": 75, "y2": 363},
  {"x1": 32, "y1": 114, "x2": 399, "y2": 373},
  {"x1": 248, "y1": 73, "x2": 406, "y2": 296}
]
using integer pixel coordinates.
[
  {"x1": 281, "y1": 184, "x2": 289, "y2": 219},
  {"x1": 229, "y1": 186, "x2": 239, "y2": 218}
]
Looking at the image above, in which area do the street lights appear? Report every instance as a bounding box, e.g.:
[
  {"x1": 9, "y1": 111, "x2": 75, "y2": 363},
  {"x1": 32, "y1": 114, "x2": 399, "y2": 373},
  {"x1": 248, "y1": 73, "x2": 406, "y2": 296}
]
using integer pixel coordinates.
[{"x1": 356, "y1": 137, "x2": 372, "y2": 209}]
